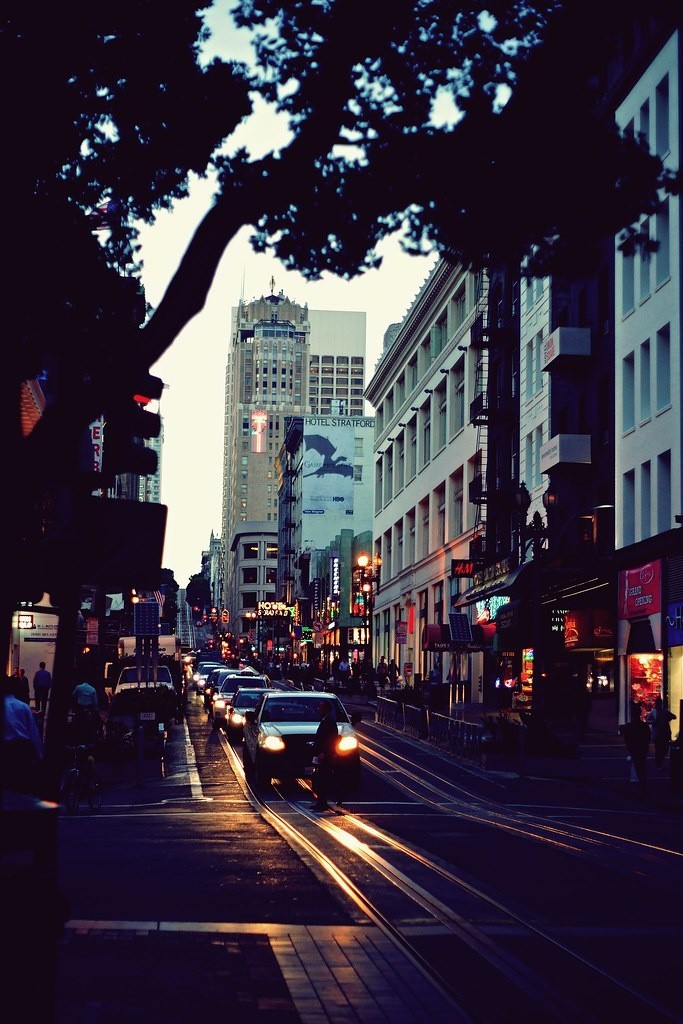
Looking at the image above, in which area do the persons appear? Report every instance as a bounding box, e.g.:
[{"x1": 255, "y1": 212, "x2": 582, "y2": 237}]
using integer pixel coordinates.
[
  {"x1": 3, "y1": 678, "x2": 43, "y2": 792},
  {"x1": 309, "y1": 701, "x2": 345, "y2": 809},
  {"x1": 71, "y1": 675, "x2": 98, "y2": 715},
  {"x1": 9, "y1": 667, "x2": 30, "y2": 705},
  {"x1": 32, "y1": 662, "x2": 51, "y2": 716},
  {"x1": 430, "y1": 665, "x2": 450, "y2": 685},
  {"x1": 333, "y1": 657, "x2": 374, "y2": 683},
  {"x1": 624, "y1": 708, "x2": 650, "y2": 793},
  {"x1": 378, "y1": 656, "x2": 400, "y2": 688},
  {"x1": 647, "y1": 697, "x2": 676, "y2": 769}
]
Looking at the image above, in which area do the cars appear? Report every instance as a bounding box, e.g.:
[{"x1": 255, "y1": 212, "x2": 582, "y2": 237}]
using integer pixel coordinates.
[
  {"x1": 243, "y1": 692, "x2": 363, "y2": 789},
  {"x1": 112, "y1": 666, "x2": 182, "y2": 707},
  {"x1": 209, "y1": 674, "x2": 268, "y2": 729},
  {"x1": 225, "y1": 688, "x2": 286, "y2": 741},
  {"x1": 192, "y1": 651, "x2": 241, "y2": 709}
]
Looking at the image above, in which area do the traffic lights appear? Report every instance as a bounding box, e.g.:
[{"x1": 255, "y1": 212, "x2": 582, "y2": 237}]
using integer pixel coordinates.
[
  {"x1": 115, "y1": 369, "x2": 162, "y2": 479},
  {"x1": 194, "y1": 606, "x2": 201, "y2": 618}
]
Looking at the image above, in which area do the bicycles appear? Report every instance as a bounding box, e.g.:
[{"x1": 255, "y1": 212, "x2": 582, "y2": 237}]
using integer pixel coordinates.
[{"x1": 60, "y1": 744, "x2": 103, "y2": 816}]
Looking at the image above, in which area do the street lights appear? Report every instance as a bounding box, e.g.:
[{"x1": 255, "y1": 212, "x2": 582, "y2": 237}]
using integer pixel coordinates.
[
  {"x1": 520, "y1": 500, "x2": 615, "y2": 783},
  {"x1": 358, "y1": 554, "x2": 369, "y2": 692}
]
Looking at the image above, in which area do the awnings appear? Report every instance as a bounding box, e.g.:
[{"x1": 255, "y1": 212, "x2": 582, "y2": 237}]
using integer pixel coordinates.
[{"x1": 422, "y1": 624, "x2": 496, "y2": 653}]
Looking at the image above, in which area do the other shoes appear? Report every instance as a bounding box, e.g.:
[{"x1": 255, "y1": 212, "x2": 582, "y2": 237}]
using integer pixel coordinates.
[
  {"x1": 310, "y1": 800, "x2": 328, "y2": 810},
  {"x1": 657, "y1": 767, "x2": 664, "y2": 771}
]
